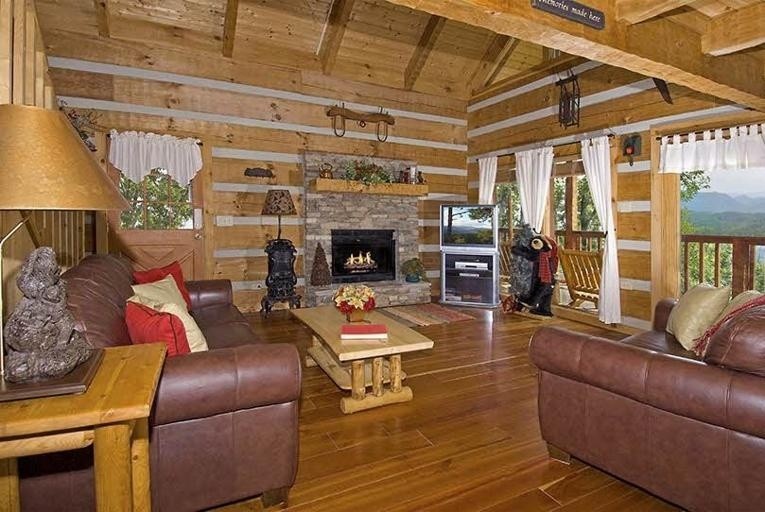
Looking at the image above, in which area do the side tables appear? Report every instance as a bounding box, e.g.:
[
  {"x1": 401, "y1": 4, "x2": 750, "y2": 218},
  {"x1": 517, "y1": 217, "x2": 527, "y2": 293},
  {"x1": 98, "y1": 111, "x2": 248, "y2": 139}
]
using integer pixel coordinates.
[{"x1": 0, "y1": 345, "x2": 168, "y2": 484}]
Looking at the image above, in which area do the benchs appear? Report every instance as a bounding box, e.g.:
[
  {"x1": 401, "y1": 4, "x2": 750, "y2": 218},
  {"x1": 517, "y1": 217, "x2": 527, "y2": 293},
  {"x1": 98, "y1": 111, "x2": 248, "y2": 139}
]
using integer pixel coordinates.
[{"x1": 497, "y1": 243, "x2": 606, "y2": 311}]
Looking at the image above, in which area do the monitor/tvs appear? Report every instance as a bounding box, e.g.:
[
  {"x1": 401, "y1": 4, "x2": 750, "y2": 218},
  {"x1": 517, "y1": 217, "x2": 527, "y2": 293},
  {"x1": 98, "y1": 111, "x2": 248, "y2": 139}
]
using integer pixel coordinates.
[{"x1": 438, "y1": 204, "x2": 498, "y2": 254}]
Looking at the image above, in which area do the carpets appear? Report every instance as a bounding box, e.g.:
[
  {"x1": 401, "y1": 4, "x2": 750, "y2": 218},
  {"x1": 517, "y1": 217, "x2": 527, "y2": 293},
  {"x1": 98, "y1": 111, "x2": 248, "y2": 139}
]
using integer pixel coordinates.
[{"x1": 377, "y1": 301, "x2": 477, "y2": 328}]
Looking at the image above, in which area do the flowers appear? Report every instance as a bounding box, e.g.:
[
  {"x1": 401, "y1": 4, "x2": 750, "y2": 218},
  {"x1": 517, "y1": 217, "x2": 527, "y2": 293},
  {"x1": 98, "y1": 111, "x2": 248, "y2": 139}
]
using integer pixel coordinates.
[{"x1": 335, "y1": 285, "x2": 373, "y2": 311}]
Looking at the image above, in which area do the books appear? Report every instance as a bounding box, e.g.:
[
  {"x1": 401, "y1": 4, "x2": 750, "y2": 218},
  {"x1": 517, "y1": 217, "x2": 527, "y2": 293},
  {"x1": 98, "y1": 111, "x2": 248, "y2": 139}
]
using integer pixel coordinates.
[{"x1": 340, "y1": 324, "x2": 389, "y2": 340}]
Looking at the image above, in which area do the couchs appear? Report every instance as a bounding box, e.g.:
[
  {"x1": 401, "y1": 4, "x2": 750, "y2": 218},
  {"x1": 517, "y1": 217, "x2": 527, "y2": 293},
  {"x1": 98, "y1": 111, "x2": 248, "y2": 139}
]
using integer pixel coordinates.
[
  {"x1": 5, "y1": 251, "x2": 303, "y2": 509},
  {"x1": 527, "y1": 299, "x2": 765, "y2": 485}
]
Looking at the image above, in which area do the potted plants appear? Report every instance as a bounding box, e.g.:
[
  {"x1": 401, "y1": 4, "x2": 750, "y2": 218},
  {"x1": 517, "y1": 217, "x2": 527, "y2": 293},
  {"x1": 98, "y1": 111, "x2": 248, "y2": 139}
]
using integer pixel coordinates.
[{"x1": 400, "y1": 256, "x2": 430, "y2": 282}]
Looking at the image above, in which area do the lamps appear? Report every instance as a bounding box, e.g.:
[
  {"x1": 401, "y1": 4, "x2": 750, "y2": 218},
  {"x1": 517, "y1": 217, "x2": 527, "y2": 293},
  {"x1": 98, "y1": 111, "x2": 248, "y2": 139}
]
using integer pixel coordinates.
[
  {"x1": 130, "y1": 262, "x2": 195, "y2": 311},
  {"x1": 258, "y1": 189, "x2": 307, "y2": 318},
  {"x1": 127, "y1": 275, "x2": 189, "y2": 308},
  {"x1": 140, "y1": 300, "x2": 208, "y2": 352},
  {"x1": 0, "y1": 103, "x2": 135, "y2": 399}
]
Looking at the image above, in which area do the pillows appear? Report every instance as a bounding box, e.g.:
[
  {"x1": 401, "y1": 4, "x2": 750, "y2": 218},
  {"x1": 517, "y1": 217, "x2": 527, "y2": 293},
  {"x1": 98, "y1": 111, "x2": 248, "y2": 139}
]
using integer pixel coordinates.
[
  {"x1": 694, "y1": 290, "x2": 763, "y2": 359},
  {"x1": 663, "y1": 281, "x2": 730, "y2": 351},
  {"x1": 123, "y1": 301, "x2": 187, "y2": 356}
]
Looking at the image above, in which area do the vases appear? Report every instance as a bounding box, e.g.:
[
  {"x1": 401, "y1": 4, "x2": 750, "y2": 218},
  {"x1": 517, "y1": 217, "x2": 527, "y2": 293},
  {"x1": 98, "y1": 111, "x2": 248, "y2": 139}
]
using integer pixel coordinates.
[{"x1": 347, "y1": 314, "x2": 364, "y2": 322}]
